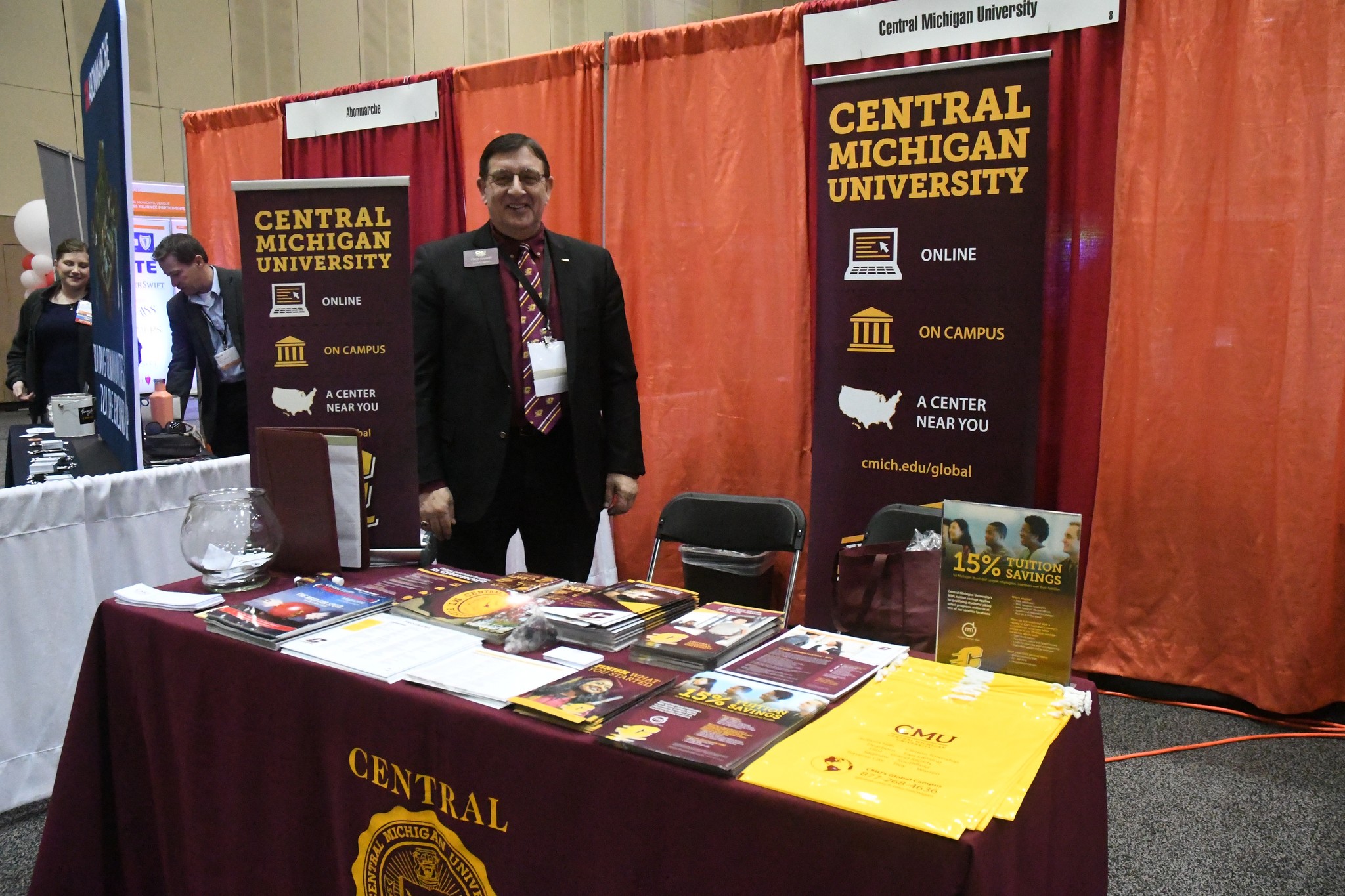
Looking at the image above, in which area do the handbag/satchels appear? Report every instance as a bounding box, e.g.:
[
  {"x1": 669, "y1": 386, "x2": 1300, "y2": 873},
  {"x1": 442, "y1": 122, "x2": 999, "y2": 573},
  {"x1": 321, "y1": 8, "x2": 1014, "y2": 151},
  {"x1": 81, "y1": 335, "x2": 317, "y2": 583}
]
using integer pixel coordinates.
[{"x1": 145, "y1": 428, "x2": 200, "y2": 456}]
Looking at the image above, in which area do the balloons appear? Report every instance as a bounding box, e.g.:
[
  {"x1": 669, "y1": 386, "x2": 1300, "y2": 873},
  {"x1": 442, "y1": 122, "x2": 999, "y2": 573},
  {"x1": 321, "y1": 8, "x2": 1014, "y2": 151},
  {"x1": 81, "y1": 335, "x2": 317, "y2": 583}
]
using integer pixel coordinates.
[
  {"x1": 21, "y1": 254, "x2": 55, "y2": 299},
  {"x1": 14, "y1": 199, "x2": 53, "y2": 258}
]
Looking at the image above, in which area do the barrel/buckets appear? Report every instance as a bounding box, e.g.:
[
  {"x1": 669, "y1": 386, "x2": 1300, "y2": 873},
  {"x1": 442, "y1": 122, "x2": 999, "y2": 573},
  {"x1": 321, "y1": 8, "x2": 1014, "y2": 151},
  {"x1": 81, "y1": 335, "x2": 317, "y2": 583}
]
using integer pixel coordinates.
[{"x1": 50, "y1": 393, "x2": 97, "y2": 437}]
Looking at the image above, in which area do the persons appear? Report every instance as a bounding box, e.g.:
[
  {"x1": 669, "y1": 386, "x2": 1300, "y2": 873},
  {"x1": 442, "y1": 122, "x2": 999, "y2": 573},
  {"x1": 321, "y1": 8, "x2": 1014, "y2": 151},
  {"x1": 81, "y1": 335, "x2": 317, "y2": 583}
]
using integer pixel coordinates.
[
  {"x1": 774, "y1": 699, "x2": 825, "y2": 727},
  {"x1": 524, "y1": 676, "x2": 623, "y2": 718},
  {"x1": 152, "y1": 233, "x2": 251, "y2": 459},
  {"x1": 5, "y1": 238, "x2": 94, "y2": 424},
  {"x1": 674, "y1": 620, "x2": 697, "y2": 629},
  {"x1": 695, "y1": 617, "x2": 754, "y2": 643},
  {"x1": 942, "y1": 515, "x2": 1081, "y2": 594},
  {"x1": 783, "y1": 632, "x2": 820, "y2": 648},
  {"x1": 722, "y1": 686, "x2": 752, "y2": 702},
  {"x1": 411, "y1": 134, "x2": 646, "y2": 584},
  {"x1": 693, "y1": 678, "x2": 717, "y2": 700},
  {"x1": 753, "y1": 690, "x2": 793, "y2": 712},
  {"x1": 807, "y1": 640, "x2": 843, "y2": 656}
]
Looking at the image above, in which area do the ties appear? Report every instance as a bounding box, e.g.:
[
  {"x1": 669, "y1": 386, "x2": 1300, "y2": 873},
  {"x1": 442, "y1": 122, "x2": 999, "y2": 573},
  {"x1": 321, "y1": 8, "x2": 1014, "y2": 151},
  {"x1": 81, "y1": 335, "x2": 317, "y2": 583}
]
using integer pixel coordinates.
[{"x1": 518, "y1": 241, "x2": 564, "y2": 434}]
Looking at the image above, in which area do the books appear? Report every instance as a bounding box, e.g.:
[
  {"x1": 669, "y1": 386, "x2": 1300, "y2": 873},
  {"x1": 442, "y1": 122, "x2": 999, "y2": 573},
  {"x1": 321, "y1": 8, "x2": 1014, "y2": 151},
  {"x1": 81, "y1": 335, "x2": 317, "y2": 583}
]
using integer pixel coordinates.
[
  {"x1": 390, "y1": 581, "x2": 559, "y2": 646},
  {"x1": 506, "y1": 600, "x2": 910, "y2": 778},
  {"x1": 489, "y1": 571, "x2": 700, "y2": 652},
  {"x1": 206, "y1": 579, "x2": 396, "y2": 651}
]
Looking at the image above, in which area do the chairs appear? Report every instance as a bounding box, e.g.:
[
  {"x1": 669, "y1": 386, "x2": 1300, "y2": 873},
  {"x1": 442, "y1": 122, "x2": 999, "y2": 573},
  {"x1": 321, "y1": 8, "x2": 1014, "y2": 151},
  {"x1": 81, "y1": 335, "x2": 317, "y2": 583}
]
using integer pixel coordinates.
[
  {"x1": 643, "y1": 493, "x2": 808, "y2": 628},
  {"x1": 863, "y1": 503, "x2": 941, "y2": 545}
]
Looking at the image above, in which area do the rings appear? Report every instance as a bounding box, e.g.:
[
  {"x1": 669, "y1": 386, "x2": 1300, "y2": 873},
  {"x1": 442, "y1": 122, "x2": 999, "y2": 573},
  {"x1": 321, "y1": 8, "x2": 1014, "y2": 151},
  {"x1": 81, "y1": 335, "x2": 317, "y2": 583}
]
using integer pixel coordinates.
[{"x1": 421, "y1": 520, "x2": 429, "y2": 527}]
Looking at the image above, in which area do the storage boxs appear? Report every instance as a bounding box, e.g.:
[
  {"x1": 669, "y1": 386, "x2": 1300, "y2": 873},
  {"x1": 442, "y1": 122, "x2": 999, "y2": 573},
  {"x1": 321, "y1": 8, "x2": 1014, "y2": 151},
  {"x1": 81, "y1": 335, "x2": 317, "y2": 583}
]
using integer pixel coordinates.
[{"x1": 29, "y1": 440, "x2": 74, "y2": 481}]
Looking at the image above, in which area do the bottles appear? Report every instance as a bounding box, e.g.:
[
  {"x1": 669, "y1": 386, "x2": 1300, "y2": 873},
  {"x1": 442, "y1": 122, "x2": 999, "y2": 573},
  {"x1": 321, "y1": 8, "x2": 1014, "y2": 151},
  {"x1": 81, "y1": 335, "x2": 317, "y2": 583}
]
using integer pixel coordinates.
[
  {"x1": 181, "y1": 487, "x2": 283, "y2": 593},
  {"x1": 149, "y1": 378, "x2": 174, "y2": 427}
]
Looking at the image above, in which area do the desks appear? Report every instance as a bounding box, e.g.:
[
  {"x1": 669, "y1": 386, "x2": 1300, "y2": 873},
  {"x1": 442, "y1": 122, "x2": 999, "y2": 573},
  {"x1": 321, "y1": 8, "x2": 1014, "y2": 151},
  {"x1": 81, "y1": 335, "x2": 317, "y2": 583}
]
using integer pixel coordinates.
[
  {"x1": 27, "y1": 563, "x2": 1109, "y2": 896},
  {"x1": 4, "y1": 421, "x2": 219, "y2": 489}
]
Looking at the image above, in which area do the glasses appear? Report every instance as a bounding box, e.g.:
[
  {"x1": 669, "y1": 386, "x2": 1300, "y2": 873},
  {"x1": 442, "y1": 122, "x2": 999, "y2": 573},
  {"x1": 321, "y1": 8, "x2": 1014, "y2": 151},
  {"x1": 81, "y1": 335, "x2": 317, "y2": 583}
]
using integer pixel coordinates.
[
  {"x1": 485, "y1": 168, "x2": 546, "y2": 185},
  {"x1": 144, "y1": 421, "x2": 187, "y2": 436}
]
